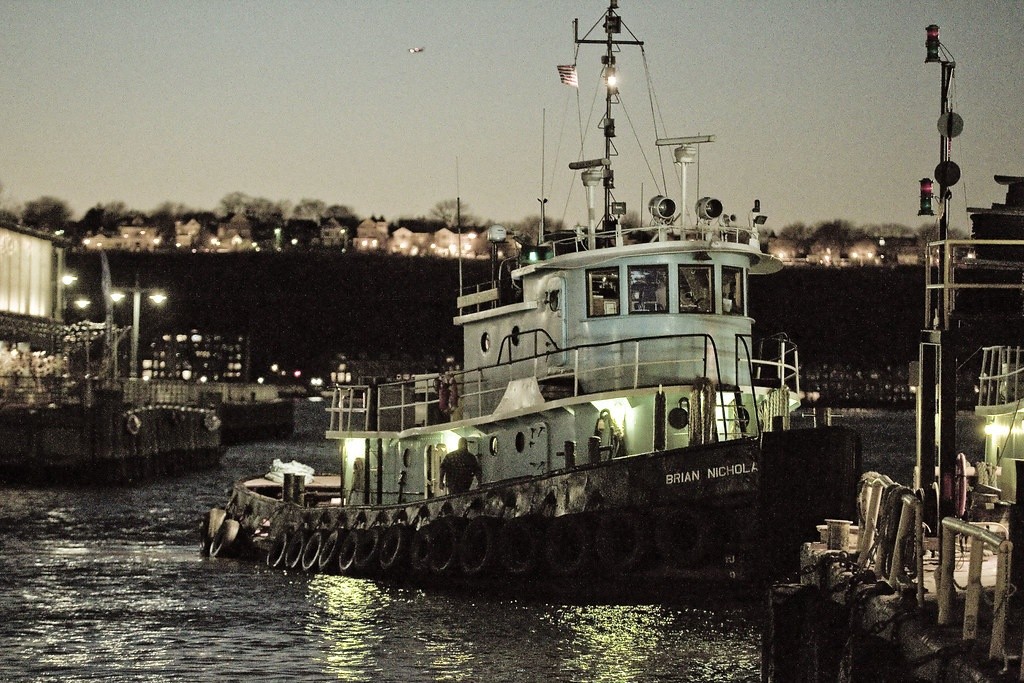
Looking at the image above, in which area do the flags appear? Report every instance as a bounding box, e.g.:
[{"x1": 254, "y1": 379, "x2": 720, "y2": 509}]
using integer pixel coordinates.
[{"x1": 556, "y1": 63, "x2": 579, "y2": 88}]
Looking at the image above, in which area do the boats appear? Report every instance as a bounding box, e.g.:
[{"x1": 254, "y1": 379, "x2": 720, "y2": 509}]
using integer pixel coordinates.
[
  {"x1": 200, "y1": 0, "x2": 875, "y2": 606},
  {"x1": 753, "y1": 21, "x2": 1023, "y2": 683}
]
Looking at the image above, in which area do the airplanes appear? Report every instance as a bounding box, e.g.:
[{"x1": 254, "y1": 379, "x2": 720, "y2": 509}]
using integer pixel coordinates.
[{"x1": 407, "y1": 46, "x2": 426, "y2": 53}]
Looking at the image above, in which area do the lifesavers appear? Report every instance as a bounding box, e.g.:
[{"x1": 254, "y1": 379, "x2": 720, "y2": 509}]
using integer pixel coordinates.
[
  {"x1": 203, "y1": 497, "x2": 744, "y2": 585},
  {"x1": 127, "y1": 414, "x2": 143, "y2": 434}
]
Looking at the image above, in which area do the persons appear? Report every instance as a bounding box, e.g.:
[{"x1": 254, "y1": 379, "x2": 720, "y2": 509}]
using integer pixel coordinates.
[{"x1": 439, "y1": 436, "x2": 483, "y2": 495}]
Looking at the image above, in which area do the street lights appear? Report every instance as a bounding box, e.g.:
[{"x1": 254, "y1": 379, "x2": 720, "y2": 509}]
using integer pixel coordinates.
[
  {"x1": 110, "y1": 287, "x2": 169, "y2": 381},
  {"x1": 73, "y1": 295, "x2": 91, "y2": 405}
]
